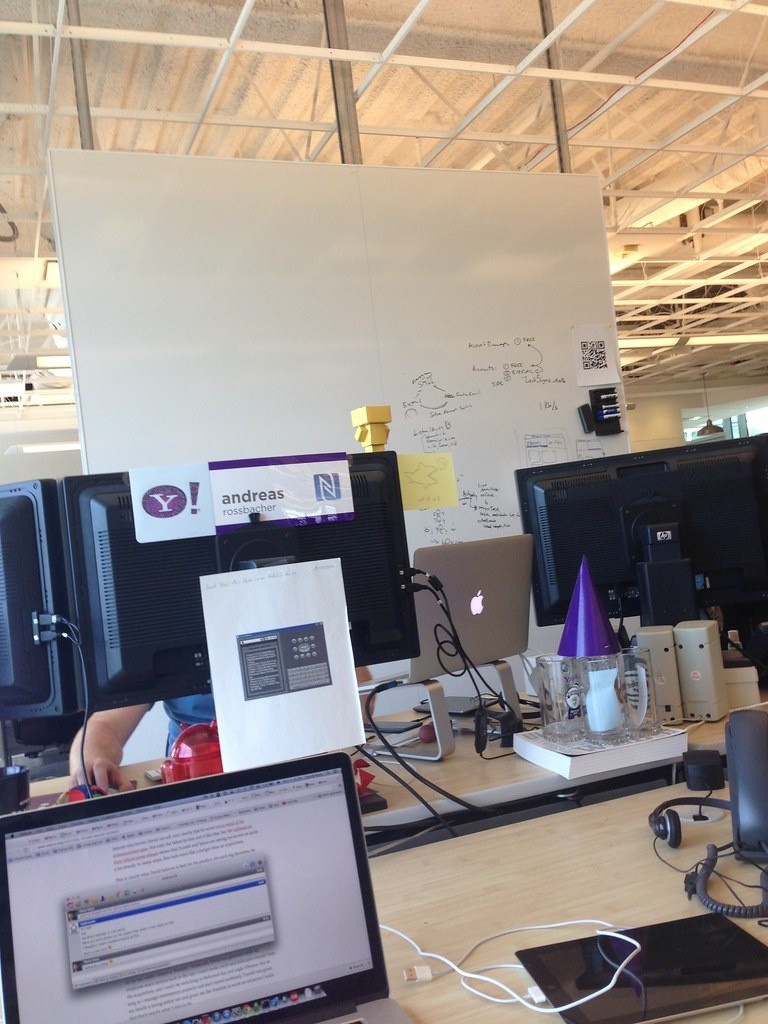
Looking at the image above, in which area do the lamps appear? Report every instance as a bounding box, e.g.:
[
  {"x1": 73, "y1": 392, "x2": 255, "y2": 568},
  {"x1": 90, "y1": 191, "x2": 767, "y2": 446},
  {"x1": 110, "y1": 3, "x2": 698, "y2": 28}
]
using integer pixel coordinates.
[{"x1": 696, "y1": 375, "x2": 724, "y2": 437}]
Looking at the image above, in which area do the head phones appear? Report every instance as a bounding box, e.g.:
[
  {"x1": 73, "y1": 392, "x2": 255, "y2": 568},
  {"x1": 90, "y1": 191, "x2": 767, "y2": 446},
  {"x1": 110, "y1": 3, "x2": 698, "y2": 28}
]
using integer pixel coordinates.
[{"x1": 648, "y1": 796, "x2": 730, "y2": 850}]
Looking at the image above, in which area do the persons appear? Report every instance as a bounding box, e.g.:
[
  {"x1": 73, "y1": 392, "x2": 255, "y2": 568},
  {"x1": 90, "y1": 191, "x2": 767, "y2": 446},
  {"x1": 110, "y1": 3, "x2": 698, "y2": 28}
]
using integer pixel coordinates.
[{"x1": 67, "y1": 666, "x2": 377, "y2": 794}]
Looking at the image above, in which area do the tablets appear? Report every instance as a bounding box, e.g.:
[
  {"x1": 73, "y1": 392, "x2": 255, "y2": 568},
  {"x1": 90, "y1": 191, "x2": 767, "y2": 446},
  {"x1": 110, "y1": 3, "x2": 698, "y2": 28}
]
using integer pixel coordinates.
[{"x1": 514, "y1": 912, "x2": 768, "y2": 1024}]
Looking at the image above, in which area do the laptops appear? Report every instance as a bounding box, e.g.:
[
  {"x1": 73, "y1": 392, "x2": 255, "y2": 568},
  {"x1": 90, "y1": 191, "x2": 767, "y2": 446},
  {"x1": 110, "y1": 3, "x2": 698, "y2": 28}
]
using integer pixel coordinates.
[
  {"x1": 0, "y1": 751, "x2": 416, "y2": 1024},
  {"x1": 357, "y1": 534, "x2": 535, "y2": 691}
]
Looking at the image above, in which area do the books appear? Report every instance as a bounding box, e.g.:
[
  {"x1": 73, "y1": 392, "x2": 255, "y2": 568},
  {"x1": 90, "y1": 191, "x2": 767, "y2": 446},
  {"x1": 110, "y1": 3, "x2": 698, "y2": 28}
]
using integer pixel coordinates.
[{"x1": 513, "y1": 726, "x2": 687, "y2": 781}]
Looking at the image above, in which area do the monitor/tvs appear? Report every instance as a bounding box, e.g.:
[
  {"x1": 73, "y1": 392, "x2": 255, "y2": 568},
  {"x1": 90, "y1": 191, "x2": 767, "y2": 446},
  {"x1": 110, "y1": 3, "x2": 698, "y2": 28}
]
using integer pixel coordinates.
[
  {"x1": 515, "y1": 433, "x2": 768, "y2": 692},
  {"x1": 0, "y1": 451, "x2": 422, "y2": 813}
]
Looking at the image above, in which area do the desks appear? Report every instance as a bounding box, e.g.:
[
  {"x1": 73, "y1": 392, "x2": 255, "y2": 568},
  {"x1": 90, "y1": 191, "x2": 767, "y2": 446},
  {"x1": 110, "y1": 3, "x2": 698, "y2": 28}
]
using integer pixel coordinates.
[
  {"x1": 29, "y1": 672, "x2": 768, "y2": 838},
  {"x1": 365, "y1": 780, "x2": 768, "y2": 1024}
]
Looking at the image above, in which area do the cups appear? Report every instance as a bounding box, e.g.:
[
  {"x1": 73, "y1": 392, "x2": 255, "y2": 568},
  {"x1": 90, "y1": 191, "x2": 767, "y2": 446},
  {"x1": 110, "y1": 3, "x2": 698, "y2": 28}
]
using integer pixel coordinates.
[
  {"x1": 0, "y1": 765, "x2": 31, "y2": 816},
  {"x1": 535, "y1": 647, "x2": 662, "y2": 746}
]
